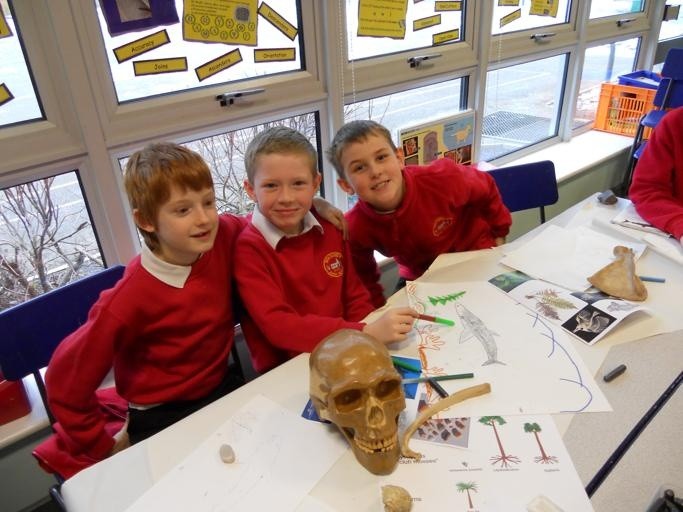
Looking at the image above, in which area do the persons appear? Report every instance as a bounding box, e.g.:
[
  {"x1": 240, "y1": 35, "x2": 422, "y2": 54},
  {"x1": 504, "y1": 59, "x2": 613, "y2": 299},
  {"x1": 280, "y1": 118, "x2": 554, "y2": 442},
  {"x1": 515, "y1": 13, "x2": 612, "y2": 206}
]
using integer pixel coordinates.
[
  {"x1": 231, "y1": 126, "x2": 421, "y2": 377},
  {"x1": 310, "y1": 332, "x2": 404, "y2": 478},
  {"x1": 327, "y1": 120, "x2": 513, "y2": 310},
  {"x1": 43, "y1": 141, "x2": 253, "y2": 461},
  {"x1": 629, "y1": 105, "x2": 682, "y2": 243}
]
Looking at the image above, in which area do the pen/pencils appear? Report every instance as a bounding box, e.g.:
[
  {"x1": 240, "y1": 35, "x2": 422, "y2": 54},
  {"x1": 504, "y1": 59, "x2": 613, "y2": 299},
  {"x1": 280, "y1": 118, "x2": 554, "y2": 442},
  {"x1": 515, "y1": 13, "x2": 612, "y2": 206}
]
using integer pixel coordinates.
[
  {"x1": 392, "y1": 358, "x2": 474, "y2": 398},
  {"x1": 640, "y1": 276, "x2": 666, "y2": 282},
  {"x1": 413, "y1": 313, "x2": 455, "y2": 326}
]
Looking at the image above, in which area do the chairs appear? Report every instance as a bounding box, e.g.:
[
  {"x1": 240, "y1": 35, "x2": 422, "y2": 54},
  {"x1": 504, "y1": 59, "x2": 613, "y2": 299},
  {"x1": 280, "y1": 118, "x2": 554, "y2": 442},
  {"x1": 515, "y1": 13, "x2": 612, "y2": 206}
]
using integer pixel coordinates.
[
  {"x1": 1, "y1": 263, "x2": 130, "y2": 510},
  {"x1": 621, "y1": 49, "x2": 683, "y2": 190},
  {"x1": 485, "y1": 159, "x2": 560, "y2": 224}
]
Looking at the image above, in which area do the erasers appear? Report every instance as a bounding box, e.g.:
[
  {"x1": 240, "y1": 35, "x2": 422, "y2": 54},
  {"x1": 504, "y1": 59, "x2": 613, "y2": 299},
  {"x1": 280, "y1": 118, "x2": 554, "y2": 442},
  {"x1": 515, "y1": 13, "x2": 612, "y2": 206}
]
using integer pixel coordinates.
[{"x1": 604, "y1": 365, "x2": 626, "y2": 382}]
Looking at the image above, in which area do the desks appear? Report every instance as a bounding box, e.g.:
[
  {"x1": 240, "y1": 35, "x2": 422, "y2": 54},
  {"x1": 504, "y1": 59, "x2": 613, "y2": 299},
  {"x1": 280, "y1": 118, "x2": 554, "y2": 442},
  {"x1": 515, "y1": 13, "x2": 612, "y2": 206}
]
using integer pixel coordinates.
[{"x1": 53, "y1": 189, "x2": 683, "y2": 512}]
